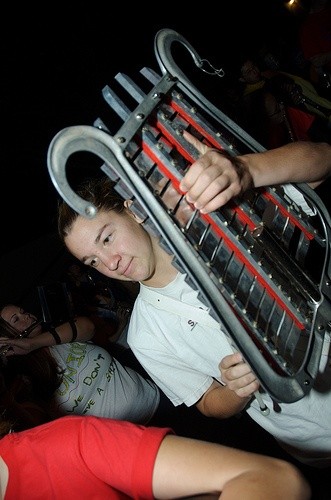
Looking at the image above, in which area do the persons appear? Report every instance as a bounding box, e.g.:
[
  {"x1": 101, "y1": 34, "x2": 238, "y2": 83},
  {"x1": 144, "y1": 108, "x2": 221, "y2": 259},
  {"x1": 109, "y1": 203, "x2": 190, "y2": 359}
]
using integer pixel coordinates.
[
  {"x1": 299, "y1": 0, "x2": 331, "y2": 85},
  {"x1": 81, "y1": 306, "x2": 130, "y2": 349},
  {"x1": 68, "y1": 261, "x2": 125, "y2": 311},
  {"x1": 249, "y1": 84, "x2": 331, "y2": 142},
  {"x1": 1, "y1": 415, "x2": 313, "y2": 500},
  {"x1": 56, "y1": 141, "x2": 331, "y2": 461},
  {"x1": 0, "y1": 304, "x2": 202, "y2": 438},
  {"x1": 235, "y1": 60, "x2": 331, "y2": 121}
]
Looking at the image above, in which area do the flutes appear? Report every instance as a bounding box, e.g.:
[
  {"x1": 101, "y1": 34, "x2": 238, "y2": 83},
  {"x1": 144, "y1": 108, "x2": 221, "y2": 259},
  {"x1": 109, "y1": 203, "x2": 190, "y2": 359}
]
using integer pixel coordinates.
[
  {"x1": 0, "y1": 318, "x2": 44, "y2": 356},
  {"x1": 257, "y1": 73, "x2": 331, "y2": 119},
  {"x1": 279, "y1": 102, "x2": 295, "y2": 142}
]
